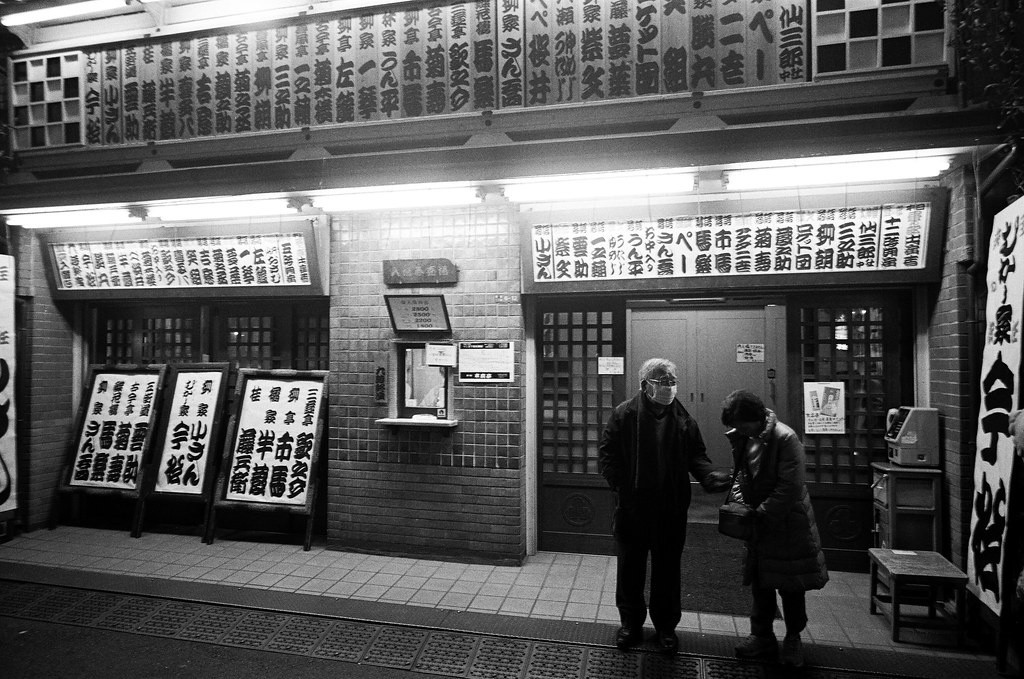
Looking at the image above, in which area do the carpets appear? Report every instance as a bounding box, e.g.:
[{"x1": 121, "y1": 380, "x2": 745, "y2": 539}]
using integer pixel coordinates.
[{"x1": 644, "y1": 521, "x2": 782, "y2": 621}]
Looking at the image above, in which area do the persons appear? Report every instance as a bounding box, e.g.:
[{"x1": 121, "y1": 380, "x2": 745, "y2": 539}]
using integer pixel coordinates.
[
  {"x1": 598, "y1": 356, "x2": 732, "y2": 656},
  {"x1": 722, "y1": 387, "x2": 829, "y2": 671}
]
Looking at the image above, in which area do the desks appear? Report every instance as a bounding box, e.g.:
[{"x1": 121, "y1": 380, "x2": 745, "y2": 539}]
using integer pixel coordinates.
[{"x1": 869, "y1": 547, "x2": 970, "y2": 648}]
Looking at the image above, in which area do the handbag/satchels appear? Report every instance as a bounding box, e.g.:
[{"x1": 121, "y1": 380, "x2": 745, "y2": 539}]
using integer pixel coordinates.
[{"x1": 717, "y1": 499, "x2": 763, "y2": 543}]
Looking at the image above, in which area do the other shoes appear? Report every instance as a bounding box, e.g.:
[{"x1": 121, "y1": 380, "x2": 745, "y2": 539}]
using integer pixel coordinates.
[
  {"x1": 614, "y1": 625, "x2": 644, "y2": 649},
  {"x1": 657, "y1": 630, "x2": 678, "y2": 653},
  {"x1": 734, "y1": 631, "x2": 778, "y2": 656},
  {"x1": 781, "y1": 632, "x2": 804, "y2": 668}
]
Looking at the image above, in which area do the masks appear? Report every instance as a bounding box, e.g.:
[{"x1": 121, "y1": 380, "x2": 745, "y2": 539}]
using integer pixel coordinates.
[{"x1": 643, "y1": 380, "x2": 677, "y2": 406}]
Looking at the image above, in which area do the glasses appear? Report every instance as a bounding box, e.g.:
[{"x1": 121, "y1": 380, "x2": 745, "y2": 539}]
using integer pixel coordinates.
[{"x1": 649, "y1": 378, "x2": 676, "y2": 387}]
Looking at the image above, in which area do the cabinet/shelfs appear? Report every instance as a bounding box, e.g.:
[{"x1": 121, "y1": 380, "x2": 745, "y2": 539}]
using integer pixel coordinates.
[{"x1": 871, "y1": 462, "x2": 944, "y2": 605}]
